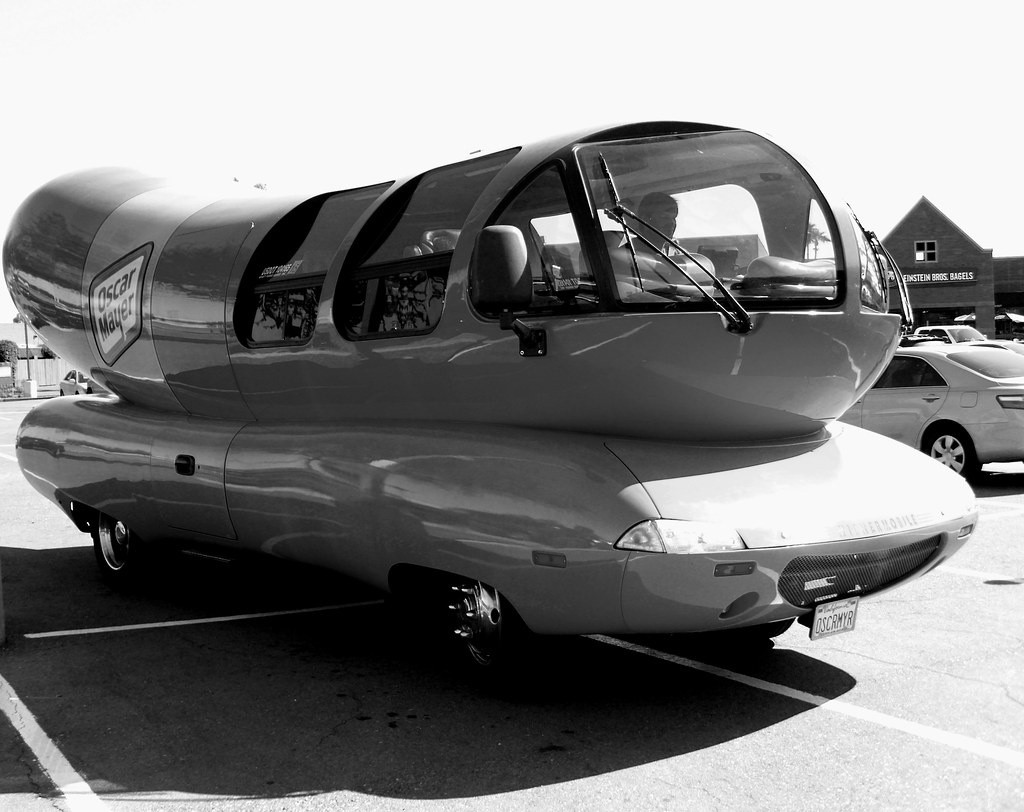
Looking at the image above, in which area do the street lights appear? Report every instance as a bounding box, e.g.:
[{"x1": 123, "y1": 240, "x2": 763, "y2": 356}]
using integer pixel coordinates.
[{"x1": 13, "y1": 312, "x2": 31, "y2": 381}]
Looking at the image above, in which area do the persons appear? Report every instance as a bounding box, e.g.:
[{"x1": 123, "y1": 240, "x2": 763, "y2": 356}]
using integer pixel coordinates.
[{"x1": 613, "y1": 194, "x2": 693, "y2": 257}]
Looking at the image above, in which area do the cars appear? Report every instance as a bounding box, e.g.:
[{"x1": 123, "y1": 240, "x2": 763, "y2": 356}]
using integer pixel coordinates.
[
  {"x1": 836, "y1": 325, "x2": 1024, "y2": 486},
  {"x1": 58, "y1": 369, "x2": 91, "y2": 396}
]
